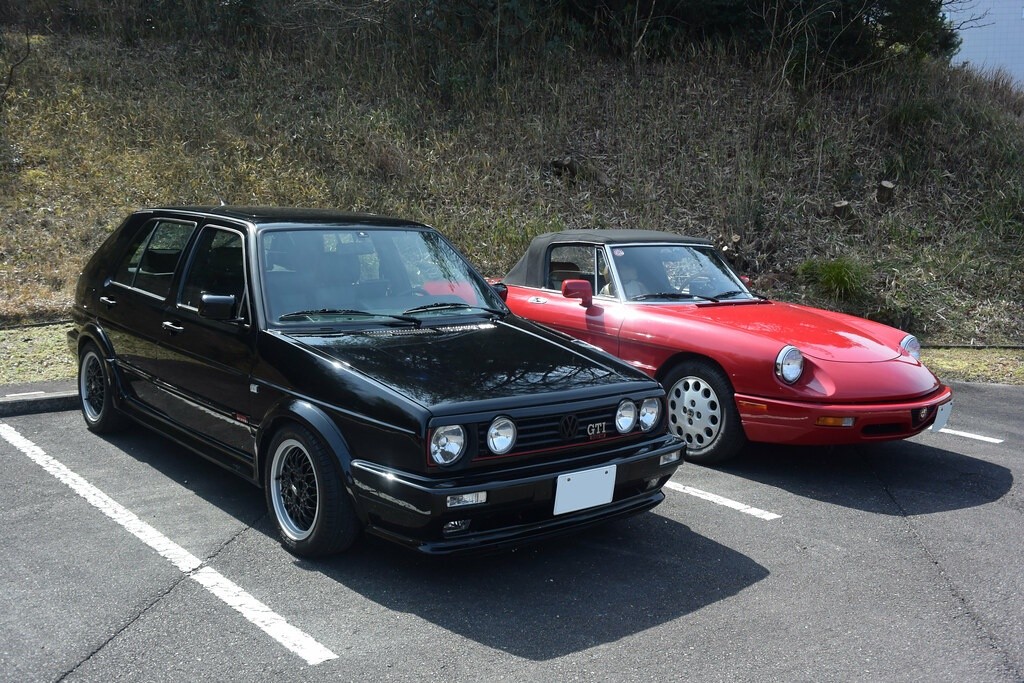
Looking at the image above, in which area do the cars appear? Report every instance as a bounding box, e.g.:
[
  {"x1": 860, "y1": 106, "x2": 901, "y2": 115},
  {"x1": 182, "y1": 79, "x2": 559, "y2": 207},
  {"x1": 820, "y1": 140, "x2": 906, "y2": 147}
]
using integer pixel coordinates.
[{"x1": 65, "y1": 207, "x2": 688, "y2": 562}]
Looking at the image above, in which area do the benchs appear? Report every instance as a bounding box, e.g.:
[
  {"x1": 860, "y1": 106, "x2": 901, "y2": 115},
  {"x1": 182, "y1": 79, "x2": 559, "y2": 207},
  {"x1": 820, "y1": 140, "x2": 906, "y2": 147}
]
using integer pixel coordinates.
[
  {"x1": 140, "y1": 244, "x2": 241, "y2": 294},
  {"x1": 548, "y1": 270, "x2": 608, "y2": 296}
]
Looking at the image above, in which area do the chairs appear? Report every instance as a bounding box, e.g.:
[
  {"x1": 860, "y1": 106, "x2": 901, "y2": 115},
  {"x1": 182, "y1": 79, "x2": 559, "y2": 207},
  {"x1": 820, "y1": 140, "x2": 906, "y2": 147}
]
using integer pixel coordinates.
[{"x1": 603, "y1": 262, "x2": 649, "y2": 300}]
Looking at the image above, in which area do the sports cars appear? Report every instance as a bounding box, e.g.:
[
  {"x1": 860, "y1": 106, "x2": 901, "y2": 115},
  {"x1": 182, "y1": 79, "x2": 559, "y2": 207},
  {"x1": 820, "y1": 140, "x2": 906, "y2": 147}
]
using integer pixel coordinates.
[{"x1": 423, "y1": 226, "x2": 954, "y2": 464}]
